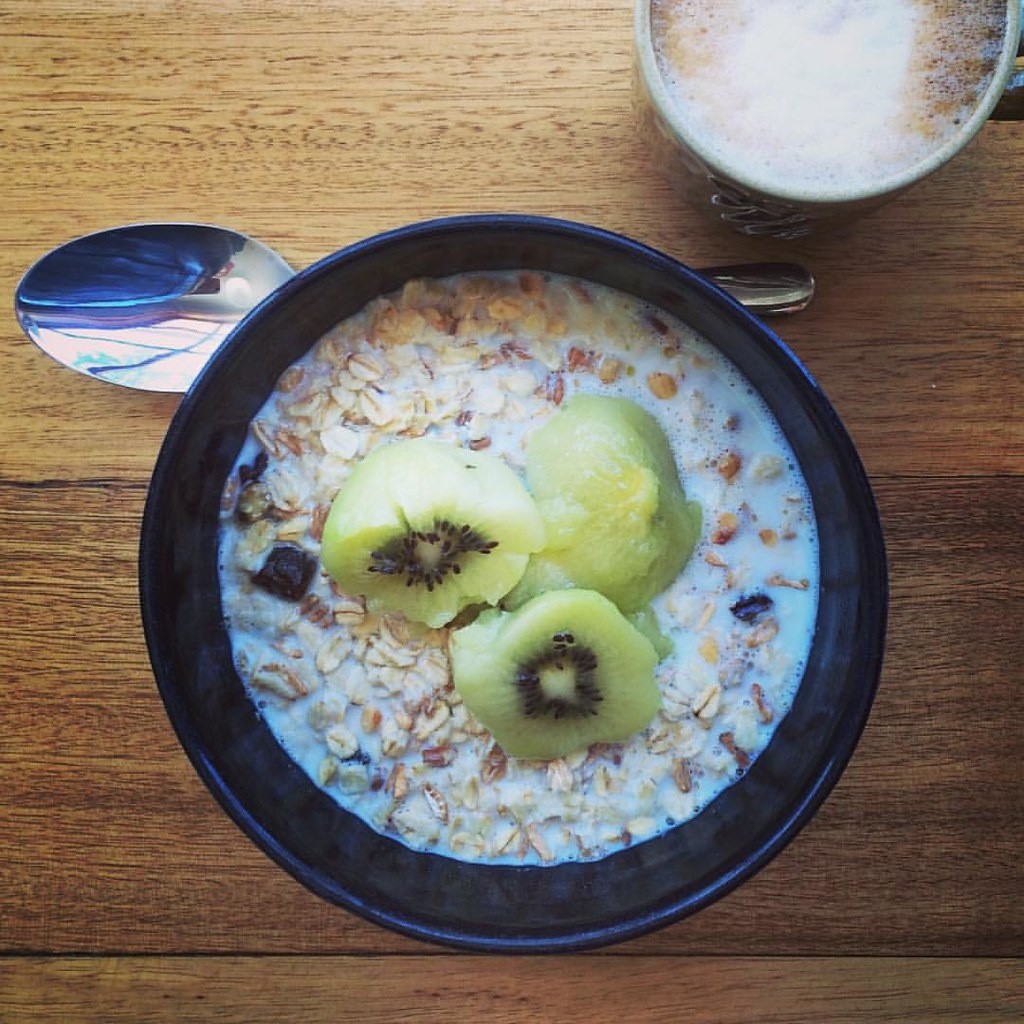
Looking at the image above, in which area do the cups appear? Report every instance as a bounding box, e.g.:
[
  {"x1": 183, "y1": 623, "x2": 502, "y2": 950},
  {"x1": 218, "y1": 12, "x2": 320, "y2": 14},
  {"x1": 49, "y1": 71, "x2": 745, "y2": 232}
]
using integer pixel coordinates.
[{"x1": 632, "y1": 1, "x2": 1023, "y2": 240}]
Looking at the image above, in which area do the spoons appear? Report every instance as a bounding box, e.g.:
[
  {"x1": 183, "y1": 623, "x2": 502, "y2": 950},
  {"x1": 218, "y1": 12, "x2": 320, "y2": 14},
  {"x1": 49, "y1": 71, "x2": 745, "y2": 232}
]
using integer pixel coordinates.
[{"x1": 14, "y1": 222, "x2": 815, "y2": 394}]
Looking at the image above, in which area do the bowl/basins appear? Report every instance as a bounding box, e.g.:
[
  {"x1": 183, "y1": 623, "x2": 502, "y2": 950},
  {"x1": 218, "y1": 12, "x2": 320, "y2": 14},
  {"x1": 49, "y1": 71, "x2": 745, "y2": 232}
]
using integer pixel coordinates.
[{"x1": 137, "y1": 211, "x2": 889, "y2": 955}]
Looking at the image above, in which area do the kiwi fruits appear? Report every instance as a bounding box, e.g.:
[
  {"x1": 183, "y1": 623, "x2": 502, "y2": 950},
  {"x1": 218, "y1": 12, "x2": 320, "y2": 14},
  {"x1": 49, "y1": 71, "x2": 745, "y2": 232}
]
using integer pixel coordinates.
[
  {"x1": 451, "y1": 394, "x2": 702, "y2": 763},
  {"x1": 317, "y1": 440, "x2": 547, "y2": 627}
]
